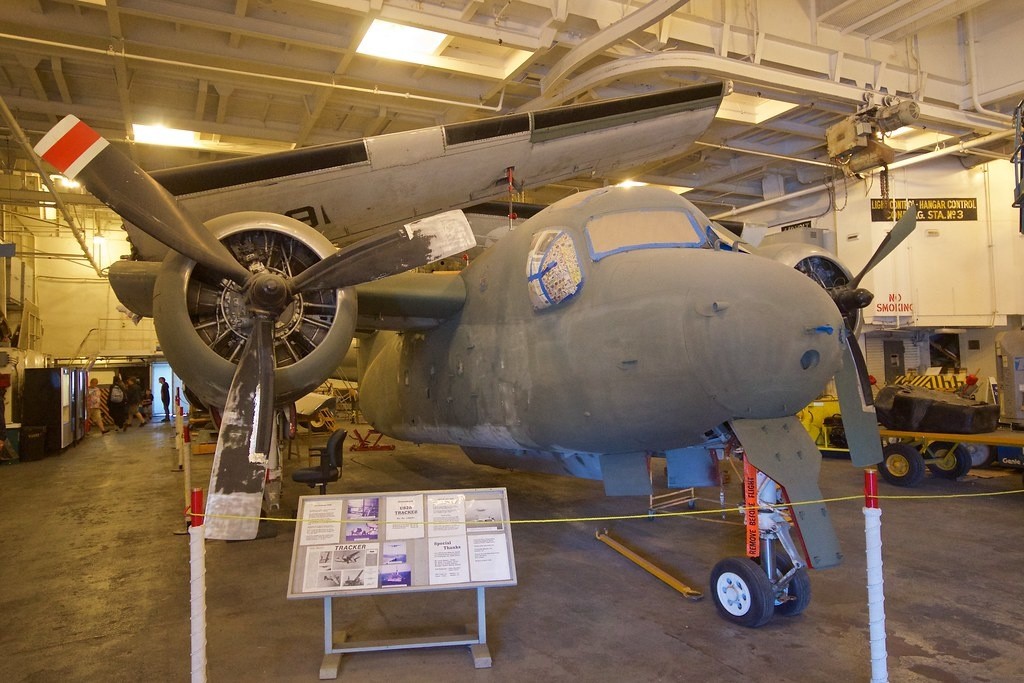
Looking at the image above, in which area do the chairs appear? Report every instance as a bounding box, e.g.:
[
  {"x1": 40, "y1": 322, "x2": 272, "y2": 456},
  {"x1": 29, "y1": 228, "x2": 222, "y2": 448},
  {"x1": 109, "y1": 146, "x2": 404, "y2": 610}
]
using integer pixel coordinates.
[{"x1": 293, "y1": 428, "x2": 348, "y2": 519}]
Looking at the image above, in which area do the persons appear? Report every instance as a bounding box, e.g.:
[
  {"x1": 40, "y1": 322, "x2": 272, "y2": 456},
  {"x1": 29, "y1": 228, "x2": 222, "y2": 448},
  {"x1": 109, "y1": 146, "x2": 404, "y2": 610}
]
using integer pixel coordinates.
[
  {"x1": 159, "y1": 377, "x2": 170, "y2": 422},
  {"x1": 107, "y1": 376, "x2": 126, "y2": 428},
  {"x1": 142, "y1": 388, "x2": 154, "y2": 420},
  {"x1": 118, "y1": 377, "x2": 147, "y2": 427},
  {"x1": 86, "y1": 378, "x2": 109, "y2": 435}
]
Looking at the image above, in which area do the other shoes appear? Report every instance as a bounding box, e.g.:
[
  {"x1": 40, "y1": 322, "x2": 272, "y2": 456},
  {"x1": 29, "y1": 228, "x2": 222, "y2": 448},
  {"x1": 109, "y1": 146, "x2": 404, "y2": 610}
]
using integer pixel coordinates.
[
  {"x1": 102, "y1": 430, "x2": 110, "y2": 434},
  {"x1": 139, "y1": 422, "x2": 147, "y2": 427},
  {"x1": 161, "y1": 419, "x2": 170, "y2": 422}
]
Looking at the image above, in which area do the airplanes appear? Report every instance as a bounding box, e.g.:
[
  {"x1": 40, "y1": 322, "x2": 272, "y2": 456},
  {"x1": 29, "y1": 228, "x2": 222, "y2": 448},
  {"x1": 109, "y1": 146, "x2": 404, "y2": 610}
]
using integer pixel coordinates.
[{"x1": 33, "y1": 79, "x2": 918, "y2": 627}]
